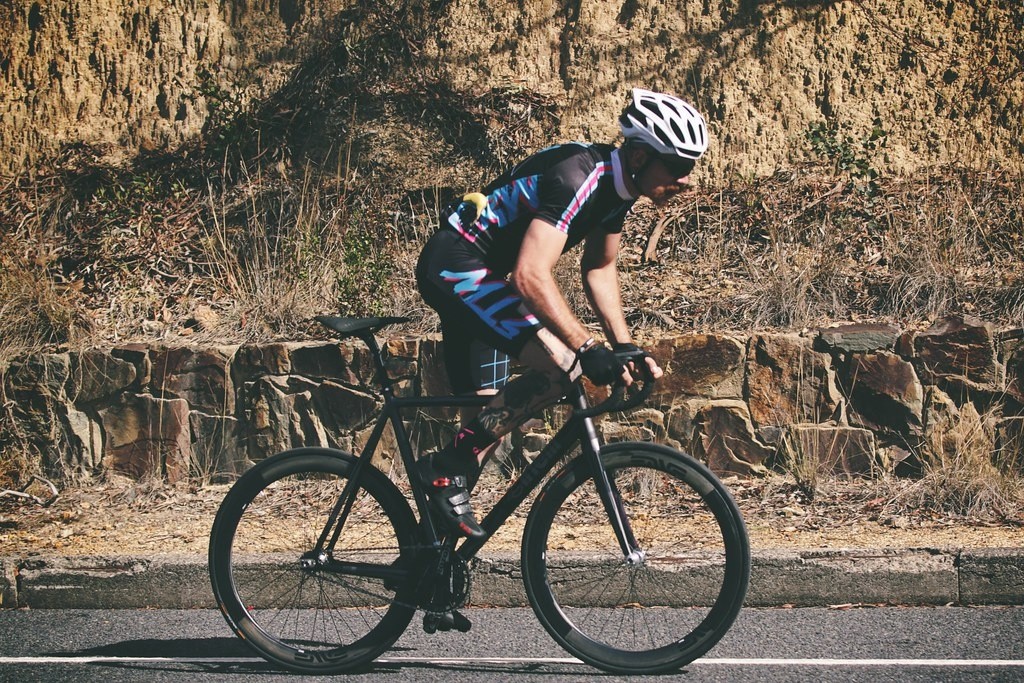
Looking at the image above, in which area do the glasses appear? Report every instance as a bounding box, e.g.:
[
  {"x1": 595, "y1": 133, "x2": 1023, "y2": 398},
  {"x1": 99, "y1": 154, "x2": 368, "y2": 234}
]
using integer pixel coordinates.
[{"x1": 653, "y1": 153, "x2": 693, "y2": 177}]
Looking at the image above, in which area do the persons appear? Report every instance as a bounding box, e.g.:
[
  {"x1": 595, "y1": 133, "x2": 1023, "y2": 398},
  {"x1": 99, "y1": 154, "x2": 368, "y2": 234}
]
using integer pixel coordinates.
[{"x1": 385, "y1": 88, "x2": 709, "y2": 633}]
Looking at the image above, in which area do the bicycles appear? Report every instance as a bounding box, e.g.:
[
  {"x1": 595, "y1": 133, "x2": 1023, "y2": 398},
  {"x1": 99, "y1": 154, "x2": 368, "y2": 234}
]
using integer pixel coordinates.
[{"x1": 207, "y1": 315, "x2": 752, "y2": 675}]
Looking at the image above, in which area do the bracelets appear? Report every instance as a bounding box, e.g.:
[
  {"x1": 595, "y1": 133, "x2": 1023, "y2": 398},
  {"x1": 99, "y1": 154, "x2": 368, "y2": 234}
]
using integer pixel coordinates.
[{"x1": 577, "y1": 337, "x2": 595, "y2": 353}]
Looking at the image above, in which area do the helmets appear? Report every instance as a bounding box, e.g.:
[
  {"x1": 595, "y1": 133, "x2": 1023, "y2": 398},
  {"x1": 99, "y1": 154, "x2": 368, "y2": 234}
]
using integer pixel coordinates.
[{"x1": 619, "y1": 88, "x2": 708, "y2": 159}]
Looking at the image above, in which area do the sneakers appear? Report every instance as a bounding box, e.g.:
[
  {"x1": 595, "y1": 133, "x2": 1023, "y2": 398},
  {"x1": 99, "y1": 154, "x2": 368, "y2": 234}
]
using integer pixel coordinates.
[
  {"x1": 391, "y1": 558, "x2": 472, "y2": 631},
  {"x1": 415, "y1": 453, "x2": 487, "y2": 538}
]
